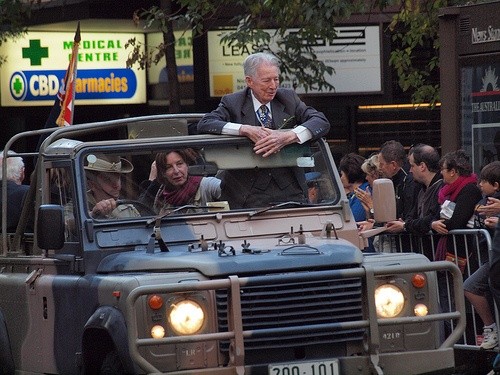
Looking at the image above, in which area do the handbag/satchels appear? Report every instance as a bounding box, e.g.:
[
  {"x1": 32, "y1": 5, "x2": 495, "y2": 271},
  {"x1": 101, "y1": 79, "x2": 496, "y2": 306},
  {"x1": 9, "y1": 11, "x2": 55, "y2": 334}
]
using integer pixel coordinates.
[{"x1": 445, "y1": 252, "x2": 467, "y2": 276}]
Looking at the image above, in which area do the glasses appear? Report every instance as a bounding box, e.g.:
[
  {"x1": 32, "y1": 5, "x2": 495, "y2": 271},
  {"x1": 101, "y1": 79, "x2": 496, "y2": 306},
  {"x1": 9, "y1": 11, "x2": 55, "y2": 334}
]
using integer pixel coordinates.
[{"x1": 440, "y1": 167, "x2": 458, "y2": 173}]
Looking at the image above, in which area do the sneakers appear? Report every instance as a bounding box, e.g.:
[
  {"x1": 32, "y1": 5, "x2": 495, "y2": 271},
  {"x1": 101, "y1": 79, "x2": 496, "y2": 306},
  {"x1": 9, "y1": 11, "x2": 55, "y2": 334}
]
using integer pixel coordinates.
[{"x1": 480, "y1": 327, "x2": 500, "y2": 350}]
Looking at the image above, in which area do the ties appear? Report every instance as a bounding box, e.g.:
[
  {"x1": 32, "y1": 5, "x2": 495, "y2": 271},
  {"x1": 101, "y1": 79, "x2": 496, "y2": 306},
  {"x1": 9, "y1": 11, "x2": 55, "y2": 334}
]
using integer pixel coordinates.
[{"x1": 260, "y1": 105, "x2": 273, "y2": 130}]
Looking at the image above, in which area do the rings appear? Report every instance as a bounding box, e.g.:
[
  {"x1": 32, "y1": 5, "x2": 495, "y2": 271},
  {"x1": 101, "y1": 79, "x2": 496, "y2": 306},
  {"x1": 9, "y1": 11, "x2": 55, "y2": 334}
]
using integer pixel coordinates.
[{"x1": 358, "y1": 195, "x2": 361, "y2": 197}]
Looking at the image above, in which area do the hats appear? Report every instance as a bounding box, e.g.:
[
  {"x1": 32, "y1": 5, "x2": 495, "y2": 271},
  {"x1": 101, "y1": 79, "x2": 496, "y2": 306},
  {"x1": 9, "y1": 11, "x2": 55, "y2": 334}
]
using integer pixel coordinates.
[
  {"x1": 83, "y1": 154, "x2": 134, "y2": 174},
  {"x1": 361, "y1": 154, "x2": 384, "y2": 177},
  {"x1": 304, "y1": 171, "x2": 321, "y2": 188}
]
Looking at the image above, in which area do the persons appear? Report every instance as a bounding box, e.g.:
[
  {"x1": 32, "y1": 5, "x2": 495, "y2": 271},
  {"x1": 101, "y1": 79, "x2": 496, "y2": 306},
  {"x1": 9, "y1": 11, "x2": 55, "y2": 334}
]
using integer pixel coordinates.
[
  {"x1": 384, "y1": 144, "x2": 445, "y2": 262},
  {"x1": 153, "y1": 147, "x2": 222, "y2": 214},
  {"x1": 355, "y1": 141, "x2": 422, "y2": 253},
  {"x1": 338, "y1": 153, "x2": 375, "y2": 252},
  {"x1": 464, "y1": 161, "x2": 500, "y2": 375},
  {"x1": 197, "y1": 53, "x2": 330, "y2": 210},
  {"x1": 353, "y1": 154, "x2": 397, "y2": 253},
  {"x1": 64, "y1": 155, "x2": 141, "y2": 242},
  {"x1": 428, "y1": 151, "x2": 483, "y2": 344},
  {"x1": 0, "y1": 150, "x2": 30, "y2": 234}
]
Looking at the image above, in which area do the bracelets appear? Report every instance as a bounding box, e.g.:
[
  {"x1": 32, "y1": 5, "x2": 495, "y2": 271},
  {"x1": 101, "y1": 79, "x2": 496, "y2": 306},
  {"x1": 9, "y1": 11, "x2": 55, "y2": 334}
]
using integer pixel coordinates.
[{"x1": 403, "y1": 222, "x2": 406, "y2": 231}]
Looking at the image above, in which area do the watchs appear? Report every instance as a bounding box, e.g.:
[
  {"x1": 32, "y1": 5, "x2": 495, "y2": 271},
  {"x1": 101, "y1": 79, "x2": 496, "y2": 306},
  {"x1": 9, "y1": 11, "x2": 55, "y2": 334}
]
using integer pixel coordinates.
[{"x1": 369, "y1": 208, "x2": 374, "y2": 214}]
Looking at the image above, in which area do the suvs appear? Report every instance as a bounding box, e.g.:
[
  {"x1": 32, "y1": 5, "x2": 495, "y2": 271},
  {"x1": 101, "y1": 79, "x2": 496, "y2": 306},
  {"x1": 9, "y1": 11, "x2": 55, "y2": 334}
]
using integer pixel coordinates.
[{"x1": 0, "y1": 113, "x2": 466, "y2": 375}]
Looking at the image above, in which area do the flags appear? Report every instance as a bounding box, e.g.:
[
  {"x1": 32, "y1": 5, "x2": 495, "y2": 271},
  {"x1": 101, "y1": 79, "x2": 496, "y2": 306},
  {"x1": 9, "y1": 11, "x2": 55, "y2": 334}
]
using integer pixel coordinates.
[{"x1": 33, "y1": 41, "x2": 79, "y2": 167}]
